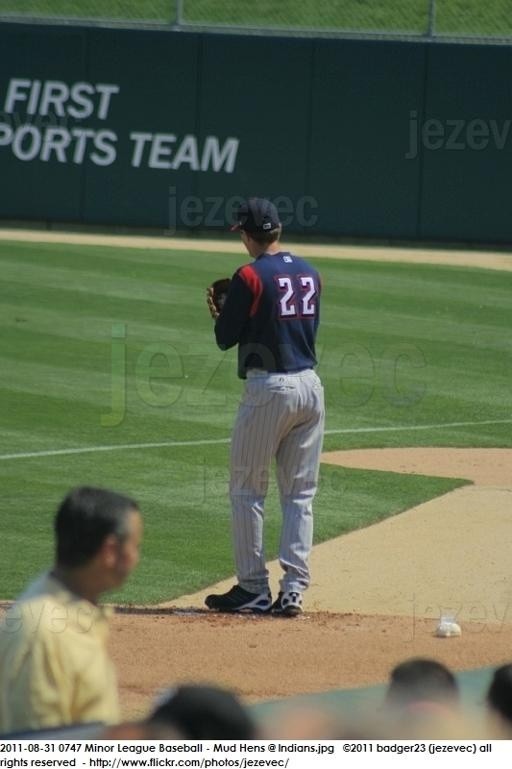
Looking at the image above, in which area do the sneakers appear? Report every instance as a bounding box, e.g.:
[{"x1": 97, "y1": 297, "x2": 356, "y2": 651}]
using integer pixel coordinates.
[
  {"x1": 269, "y1": 589, "x2": 304, "y2": 616},
  {"x1": 204, "y1": 583, "x2": 273, "y2": 618}
]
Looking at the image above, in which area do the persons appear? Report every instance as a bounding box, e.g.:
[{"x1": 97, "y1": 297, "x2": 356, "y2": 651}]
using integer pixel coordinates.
[
  {"x1": 144, "y1": 685, "x2": 259, "y2": 740},
  {"x1": 382, "y1": 658, "x2": 461, "y2": 714},
  {"x1": 485, "y1": 661, "x2": 511, "y2": 740},
  {"x1": 0, "y1": 484, "x2": 143, "y2": 732},
  {"x1": 203, "y1": 195, "x2": 326, "y2": 616}
]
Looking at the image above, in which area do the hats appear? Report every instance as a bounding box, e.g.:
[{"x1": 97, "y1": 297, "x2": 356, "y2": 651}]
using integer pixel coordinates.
[{"x1": 230, "y1": 198, "x2": 282, "y2": 233}]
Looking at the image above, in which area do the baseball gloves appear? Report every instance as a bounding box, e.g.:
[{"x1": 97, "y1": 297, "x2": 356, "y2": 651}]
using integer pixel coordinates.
[{"x1": 207, "y1": 279, "x2": 233, "y2": 320}]
